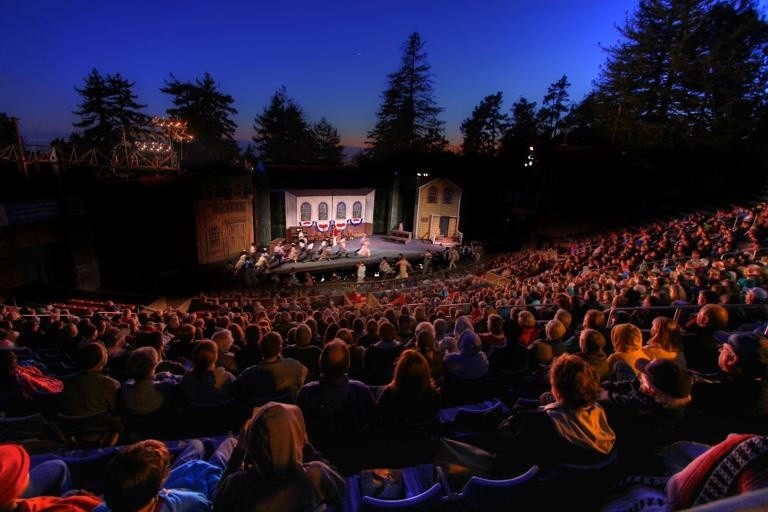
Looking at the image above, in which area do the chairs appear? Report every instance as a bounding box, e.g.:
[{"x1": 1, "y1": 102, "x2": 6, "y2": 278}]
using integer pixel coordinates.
[
  {"x1": 441, "y1": 398, "x2": 509, "y2": 434},
  {"x1": 610, "y1": 437, "x2": 768, "y2": 511},
  {"x1": 351, "y1": 464, "x2": 438, "y2": 512},
  {"x1": 514, "y1": 397, "x2": 539, "y2": 405},
  {"x1": 0, "y1": 389, "x2": 440, "y2": 443},
  {"x1": 439, "y1": 461, "x2": 540, "y2": 512},
  {"x1": 548, "y1": 444, "x2": 622, "y2": 512}
]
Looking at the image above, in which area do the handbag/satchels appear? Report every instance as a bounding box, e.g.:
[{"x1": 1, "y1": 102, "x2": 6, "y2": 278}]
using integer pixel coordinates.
[{"x1": 357, "y1": 467, "x2": 405, "y2": 502}]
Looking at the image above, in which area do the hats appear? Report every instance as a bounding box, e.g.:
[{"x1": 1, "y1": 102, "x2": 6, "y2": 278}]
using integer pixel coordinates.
[
  {"x1": 742, "y1": 286, "x2": 768, "y2": 303},
  {"x1": 635, "y1": 357, "x2": 692, "y2": 398},
  {"x1": 711, "y1": 326, "x2": 768, "y2": 377}
]
